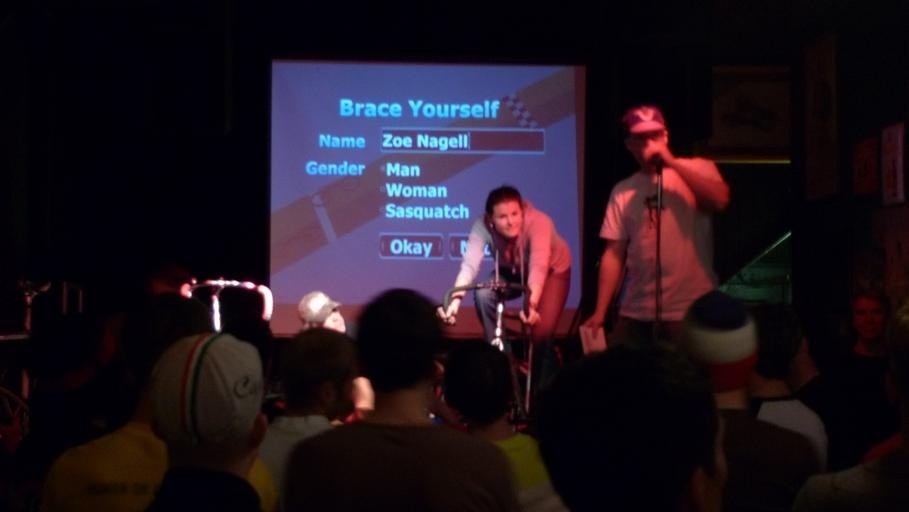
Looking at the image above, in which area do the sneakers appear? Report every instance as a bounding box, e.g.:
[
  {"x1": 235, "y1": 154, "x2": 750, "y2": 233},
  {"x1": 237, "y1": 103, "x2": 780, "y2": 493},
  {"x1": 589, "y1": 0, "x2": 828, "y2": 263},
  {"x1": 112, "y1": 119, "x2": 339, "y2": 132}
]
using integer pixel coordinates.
[{"x1": 520, "y1": 361, "x2": 528, "y2": 375}]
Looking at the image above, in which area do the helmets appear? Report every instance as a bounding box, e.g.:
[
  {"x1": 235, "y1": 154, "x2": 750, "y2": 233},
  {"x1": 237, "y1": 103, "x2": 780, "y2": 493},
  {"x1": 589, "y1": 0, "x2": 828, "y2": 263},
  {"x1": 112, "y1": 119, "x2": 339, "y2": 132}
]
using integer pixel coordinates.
[{"x1": 684, "y1": 291, "x2": 758, "y2": 392}]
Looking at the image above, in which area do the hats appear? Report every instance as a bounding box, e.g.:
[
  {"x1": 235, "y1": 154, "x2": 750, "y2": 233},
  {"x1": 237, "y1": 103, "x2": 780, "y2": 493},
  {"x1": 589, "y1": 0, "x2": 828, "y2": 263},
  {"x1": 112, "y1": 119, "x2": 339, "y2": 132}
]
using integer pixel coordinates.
[
  {"x1": 623, "y1": 107, "x2": 666, "y2": 134},
  {"x1": 298, "y1": 291, "x2": 343, "y2": 322},
  {"x1": 151, "y1": 333, "x2": 264, "y2": 444}
]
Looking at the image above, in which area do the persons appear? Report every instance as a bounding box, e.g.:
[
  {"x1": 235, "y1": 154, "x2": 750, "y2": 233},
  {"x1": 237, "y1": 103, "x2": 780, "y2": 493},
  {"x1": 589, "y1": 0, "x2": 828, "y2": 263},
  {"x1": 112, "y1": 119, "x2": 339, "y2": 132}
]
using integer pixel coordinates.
[
  {"x1": 583, "y1": 105, "x2": 732, "y2": 354},
  {"x1": 1, "y1": 280, "x2": 907, "y2": 510},
  {"x1": 435, "y1": 183, "x2": 573, "y2": 425}
]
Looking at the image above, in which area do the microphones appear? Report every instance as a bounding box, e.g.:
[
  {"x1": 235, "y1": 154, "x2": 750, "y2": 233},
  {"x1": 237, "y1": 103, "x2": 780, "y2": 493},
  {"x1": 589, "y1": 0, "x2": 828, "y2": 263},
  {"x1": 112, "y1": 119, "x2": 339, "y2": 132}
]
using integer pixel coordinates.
[{"x1": 651, "y1": 153, "x2": 665, "y2": 172}]
